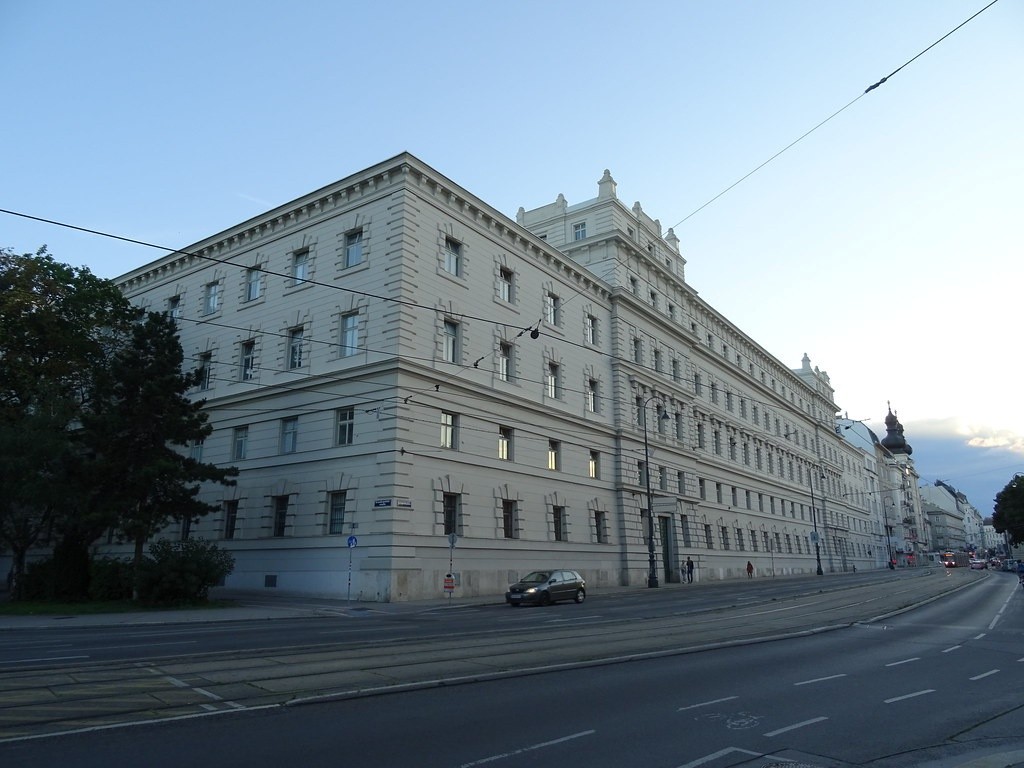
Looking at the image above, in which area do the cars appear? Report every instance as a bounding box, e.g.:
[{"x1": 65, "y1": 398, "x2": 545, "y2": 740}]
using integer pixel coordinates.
[
  {"x1": 968, "y1": 554, "x2": 1019, "y2": 573},
  {"x1": 945, "y1": 558, "x2": 958, "y2": 568}
]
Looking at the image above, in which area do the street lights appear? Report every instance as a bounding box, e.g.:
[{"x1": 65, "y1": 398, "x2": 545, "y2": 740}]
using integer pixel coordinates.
[
  {"x1": 808, "y1": 464, "x2": 826, "y2": 575},
  {"x1": 643, "y1": 396, "x2": 670, "y2": 588},
  {"x1": 883, "y1": 496, "x2": 896, "y2": 570}
]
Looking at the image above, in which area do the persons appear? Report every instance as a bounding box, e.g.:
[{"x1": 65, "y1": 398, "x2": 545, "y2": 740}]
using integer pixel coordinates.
[
  {"x1": 686, "y1": 555, "x2": 694, "y2": 583},
  {"x1": 746, "y1": 561, "x2": 753, "y2": 578},
  {"x1": 679, "y1": 560, "x2": 687, "y2": 583}
]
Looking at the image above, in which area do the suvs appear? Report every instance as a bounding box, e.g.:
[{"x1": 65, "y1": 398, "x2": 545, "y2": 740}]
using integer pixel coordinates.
[{"x1": 505, "y1": 568, "x2": 587, "y2": 608}]
